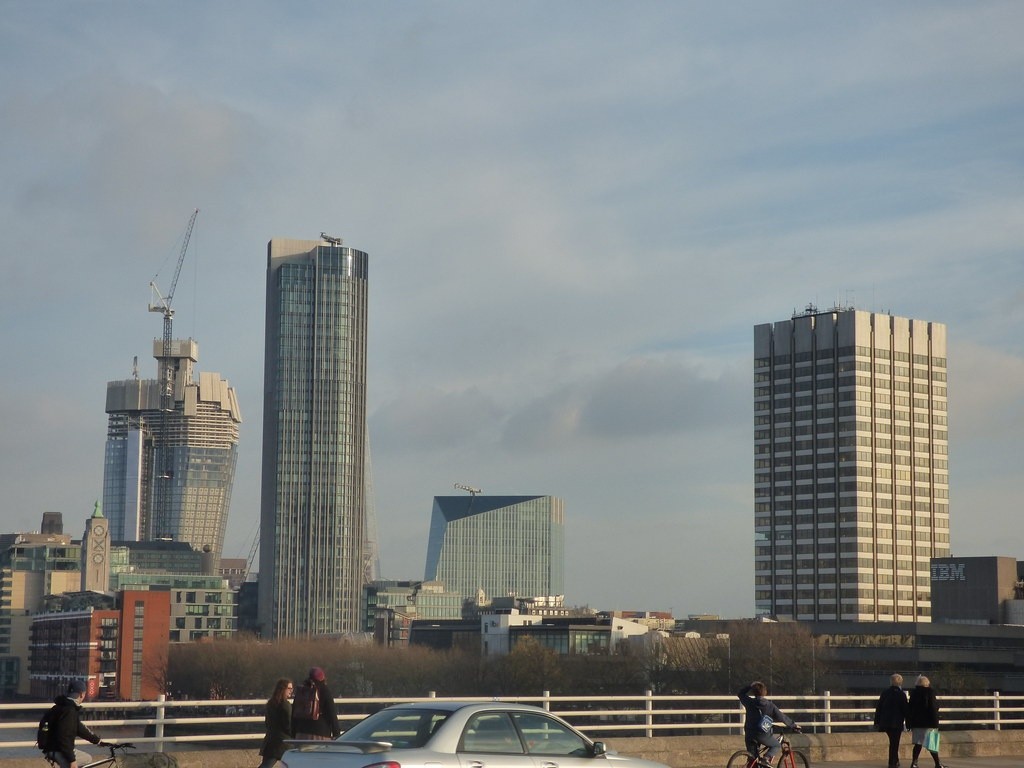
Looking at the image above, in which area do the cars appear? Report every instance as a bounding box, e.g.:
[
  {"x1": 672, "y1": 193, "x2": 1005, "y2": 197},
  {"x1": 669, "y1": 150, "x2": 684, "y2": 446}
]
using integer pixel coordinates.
[{"x1": 270, "y1": 700, "x2": 671, "y2": 768}]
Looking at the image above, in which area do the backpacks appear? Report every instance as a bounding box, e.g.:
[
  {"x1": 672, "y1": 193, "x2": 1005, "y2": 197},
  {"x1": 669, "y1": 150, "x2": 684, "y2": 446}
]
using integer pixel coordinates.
[{"x1": 293, "y1": 680, "x2": 321, "y2": 721}]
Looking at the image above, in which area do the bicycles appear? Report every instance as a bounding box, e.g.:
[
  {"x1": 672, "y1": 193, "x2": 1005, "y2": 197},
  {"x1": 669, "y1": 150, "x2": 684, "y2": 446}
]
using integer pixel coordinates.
[
  {"x1": 41, "y1": 741, "x2": 137, "y2": 768},
  {"x1": 727, "y1": 724, "x2": 809, "y2": 768}
]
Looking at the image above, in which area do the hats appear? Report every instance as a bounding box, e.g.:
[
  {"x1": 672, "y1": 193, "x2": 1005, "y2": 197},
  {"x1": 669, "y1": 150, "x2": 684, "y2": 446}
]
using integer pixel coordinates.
[
  {"x1": 67, "y1": 680, "x2": 87, "y2": 699},
  {"x1": 309, "y1": 667, "x2": 324, "y2": 679}
]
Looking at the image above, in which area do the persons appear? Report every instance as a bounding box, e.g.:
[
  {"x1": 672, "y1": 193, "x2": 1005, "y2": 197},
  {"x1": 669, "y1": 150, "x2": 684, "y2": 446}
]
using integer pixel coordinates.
[
  {"x1": 43, "y1": 681, "x2": 102, "y2": 768},
  {"x1": 737, "y1": 681, "x2": 801, "y2": 768},
  {"x1": 873, "y1": 674, "x2": 949, "y2": 768},
  {"x1": 257, "y1": 667, "x2": 340, "y2": 768}
]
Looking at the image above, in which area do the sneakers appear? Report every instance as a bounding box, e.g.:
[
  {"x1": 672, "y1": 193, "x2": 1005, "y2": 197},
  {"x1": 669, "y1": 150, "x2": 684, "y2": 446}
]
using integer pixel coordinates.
[{"x1": 759, "y1": 757, "x2": 773, "y2": 767}]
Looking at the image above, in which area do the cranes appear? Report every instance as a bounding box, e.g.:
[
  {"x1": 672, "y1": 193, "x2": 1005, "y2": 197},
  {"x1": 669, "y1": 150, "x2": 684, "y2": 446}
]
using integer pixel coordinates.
[{"x1": 148, "y1": 203, "x2": 201, "y2": 539}]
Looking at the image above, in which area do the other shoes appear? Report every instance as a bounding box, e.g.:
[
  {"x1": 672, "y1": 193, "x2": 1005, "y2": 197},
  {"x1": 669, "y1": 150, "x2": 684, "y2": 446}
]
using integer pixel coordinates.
[
  {"x1": 911, "y1": 762, "x2": 918, "y2": 768},
  {"x1": 934, "y1": 764, "x2": 948, "y2": 768}
]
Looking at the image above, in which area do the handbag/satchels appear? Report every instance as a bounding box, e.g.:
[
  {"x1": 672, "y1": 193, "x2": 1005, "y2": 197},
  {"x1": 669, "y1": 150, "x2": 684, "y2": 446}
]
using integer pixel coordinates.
[
  {"x1": 927, "y1": 729, "x2": 940, "y2": 752},
  {"x1": 759, "y1": 708, "x2": 776, "y2": 735}
]
